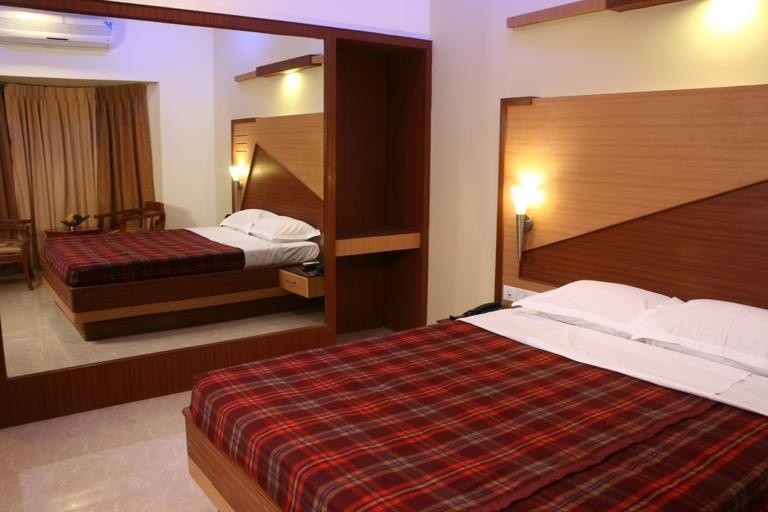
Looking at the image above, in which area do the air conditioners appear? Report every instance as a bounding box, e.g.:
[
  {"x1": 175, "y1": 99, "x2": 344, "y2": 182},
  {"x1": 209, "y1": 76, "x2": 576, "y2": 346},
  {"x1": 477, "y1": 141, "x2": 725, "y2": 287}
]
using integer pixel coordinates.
[{"x1": 0, "y1": 5, "x2": 114, "y2": 53}]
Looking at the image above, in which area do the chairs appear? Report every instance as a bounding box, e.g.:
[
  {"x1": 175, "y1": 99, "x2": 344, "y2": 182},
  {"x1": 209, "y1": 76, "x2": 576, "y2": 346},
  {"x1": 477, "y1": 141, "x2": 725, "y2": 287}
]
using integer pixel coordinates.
[
  {"x1": 94, "y1": 200, "x2": 166, "y2": 230},
  {"x1": 0, "y1": 219, "x2": 34, "y2": 292}
]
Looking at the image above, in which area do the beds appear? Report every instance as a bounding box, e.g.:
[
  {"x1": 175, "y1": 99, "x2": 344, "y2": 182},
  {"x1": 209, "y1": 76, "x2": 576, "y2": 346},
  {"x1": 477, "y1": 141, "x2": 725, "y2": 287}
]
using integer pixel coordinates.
[
  {"x1": 41, "y1": 111, "x2": 326, "y2": 341},
  {"x1": 180, "y1": 83, "x2": 768, "y2": 512}
]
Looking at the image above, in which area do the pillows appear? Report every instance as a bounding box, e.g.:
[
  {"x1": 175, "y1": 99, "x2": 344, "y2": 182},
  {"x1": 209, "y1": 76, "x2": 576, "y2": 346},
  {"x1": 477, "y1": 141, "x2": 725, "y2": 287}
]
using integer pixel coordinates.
[
  {"x1": 250, "y1": 214, "x2": 320, "y2": 242},
  {"x1": 627, "y1": 297, "x2": 768, "y2": 375},
  {"x1": 220, "y1": 209, "x2": 278, "y2": 234},
  {"x1": 510, "y1": 277, "x2": 678, "y2": 336}
]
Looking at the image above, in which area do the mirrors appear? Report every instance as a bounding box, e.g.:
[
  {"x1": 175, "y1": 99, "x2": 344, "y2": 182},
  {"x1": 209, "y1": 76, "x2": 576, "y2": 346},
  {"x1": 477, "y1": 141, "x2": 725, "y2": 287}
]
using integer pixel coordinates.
[{"x1": 0, "y1": 0, "x2": 326, "y2": 384}]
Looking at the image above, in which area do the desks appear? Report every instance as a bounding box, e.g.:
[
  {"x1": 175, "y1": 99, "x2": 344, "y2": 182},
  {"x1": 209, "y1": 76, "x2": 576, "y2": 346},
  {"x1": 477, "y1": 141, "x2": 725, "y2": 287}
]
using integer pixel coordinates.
[{"x1": 45, "y1": 229, "x2": 97, "y2": 236}]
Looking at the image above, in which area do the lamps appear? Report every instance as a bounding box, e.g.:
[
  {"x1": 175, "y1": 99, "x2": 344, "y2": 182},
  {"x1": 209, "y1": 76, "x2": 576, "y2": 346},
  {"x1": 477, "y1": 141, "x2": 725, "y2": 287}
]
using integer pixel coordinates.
[
  {"x1": 228, "y1": 165, "x2": 245, "y2": 190},
  {"x1": 515, "y1": 206, "x2": 534, "y2": 262}
]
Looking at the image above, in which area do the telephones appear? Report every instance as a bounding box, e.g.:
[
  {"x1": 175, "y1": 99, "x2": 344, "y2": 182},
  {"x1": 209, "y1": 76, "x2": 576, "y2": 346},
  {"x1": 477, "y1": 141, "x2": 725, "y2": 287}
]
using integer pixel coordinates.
[{"x1": 449, "y1": 302, "x2": 505, "y2": 321}]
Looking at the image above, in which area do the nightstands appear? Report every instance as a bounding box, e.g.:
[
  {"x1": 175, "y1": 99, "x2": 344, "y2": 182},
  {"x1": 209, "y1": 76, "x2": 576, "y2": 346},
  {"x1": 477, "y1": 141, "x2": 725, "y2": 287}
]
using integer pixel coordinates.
[{"x1": 279, "y1": 264, "x2": 325, "y2": 299}]
